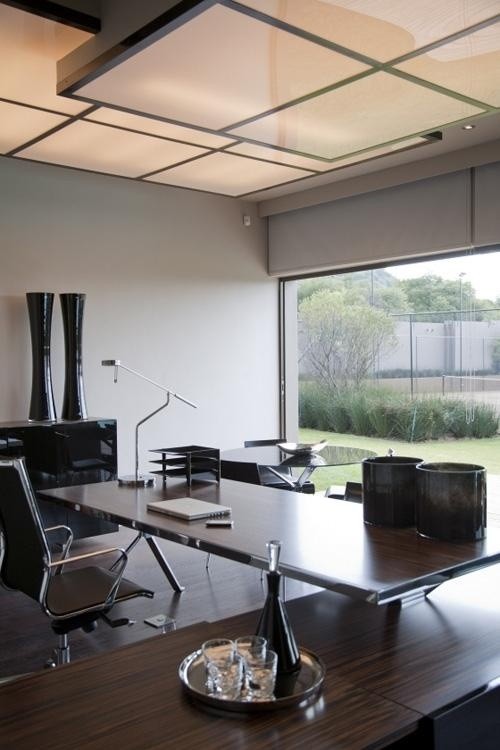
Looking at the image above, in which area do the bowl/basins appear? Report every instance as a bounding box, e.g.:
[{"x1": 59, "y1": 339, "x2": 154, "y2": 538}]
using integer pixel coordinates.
[
  {"x1": 279, "y1": 454, "x2": 327, "y2": 467},
  {"x1": 275, "y1": 439, "x2": 330, "y2": 456}
]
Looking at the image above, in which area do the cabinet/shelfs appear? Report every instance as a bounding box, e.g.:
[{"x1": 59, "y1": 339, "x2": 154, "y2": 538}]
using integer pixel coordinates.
[
  {"x1": 149, "y1": 445, "x2": 220, "y2": 488},
  {"x1": 0, "y1": 417, "x2": 119, "y2": 547}
]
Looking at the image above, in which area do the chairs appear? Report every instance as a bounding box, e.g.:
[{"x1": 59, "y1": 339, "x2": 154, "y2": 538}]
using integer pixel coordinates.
[
  {"x1": 220, "y1": 460, "x2": 264, "y2": 485},
  {"x1": 0, "y1": 455, "x2": 155, "y2": 669},
  {"x1": 244, "y1": 439, "x2": 315, "y2": 494}
]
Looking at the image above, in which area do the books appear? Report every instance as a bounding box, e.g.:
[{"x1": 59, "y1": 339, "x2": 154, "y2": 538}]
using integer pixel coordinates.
[{"x1": 147, "y1": 496, "x2": 229, "y2": 521}]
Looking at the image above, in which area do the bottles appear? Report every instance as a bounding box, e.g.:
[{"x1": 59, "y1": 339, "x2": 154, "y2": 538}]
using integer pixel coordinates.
[{"x1": 250, "y1": 538, "x2": 302, "y2": 676}]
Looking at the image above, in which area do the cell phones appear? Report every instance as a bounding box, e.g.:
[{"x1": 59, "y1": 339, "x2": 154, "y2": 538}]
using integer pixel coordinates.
[{"x1": 206, "y1": 520, "x2": 234, "y2": 527}]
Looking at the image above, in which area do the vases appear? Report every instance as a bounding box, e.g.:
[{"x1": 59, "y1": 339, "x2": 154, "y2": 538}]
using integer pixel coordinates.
[
  {"x1": 25, "y1": 292, "x2": 57, "y2": 423},
  {"x1": 59, "y1": 293, "x2": 88, "y2": 421}
]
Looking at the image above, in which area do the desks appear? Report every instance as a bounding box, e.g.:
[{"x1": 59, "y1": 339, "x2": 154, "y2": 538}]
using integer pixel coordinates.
[
  {"x1": 36, "y1": 472, "x2": 500, "y2": 606},
  {"x1": 0, "y1": 590, "x2": 500, "y2": 750}
]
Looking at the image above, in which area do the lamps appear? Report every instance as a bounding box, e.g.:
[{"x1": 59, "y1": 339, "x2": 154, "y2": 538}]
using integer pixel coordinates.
[{"x1": 101, "y1": 359, "x2": 197, "y2": 487}]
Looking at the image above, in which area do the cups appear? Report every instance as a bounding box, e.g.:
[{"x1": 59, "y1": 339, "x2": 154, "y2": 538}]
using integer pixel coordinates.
[
  {"x1": 361, "y1": 456, "x2": 427, "y2": 529},
  {"x1": 207, "y1": 651, "x2": 243, "y2": 701},
  {"x1": 243, "y1": 651, "x2": 278, "y2": 699},
  {"x1": 234, "y1": 635, "x2": 268, "y2": 669},
  {"x1": 201, "y1": 638, "x2": 236, "y2": 676},
  {"x1": 413, "y1": 462, "x2": 488, "y2": 543}
]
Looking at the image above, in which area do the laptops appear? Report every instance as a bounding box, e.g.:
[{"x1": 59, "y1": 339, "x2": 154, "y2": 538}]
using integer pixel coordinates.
[{"x1": 147, "y1": 497, "x2": 231, "y2": 520}]
